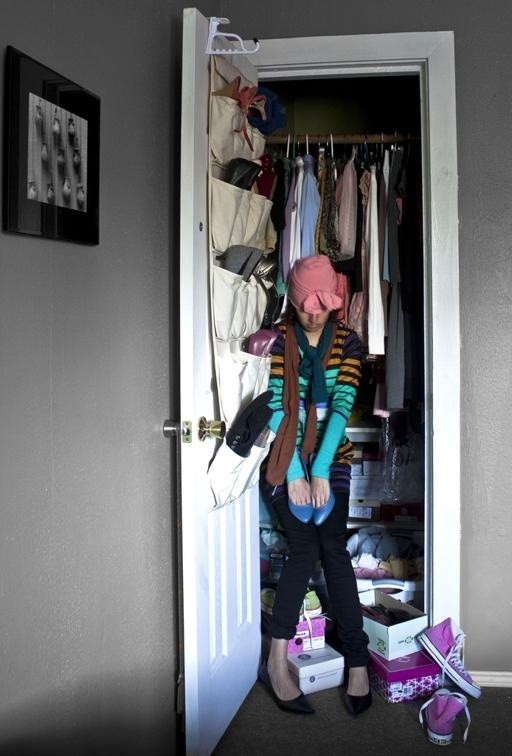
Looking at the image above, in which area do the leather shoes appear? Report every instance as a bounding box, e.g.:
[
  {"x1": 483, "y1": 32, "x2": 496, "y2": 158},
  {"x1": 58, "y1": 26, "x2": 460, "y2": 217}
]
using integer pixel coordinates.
[
  {"x1": 260, "y1": 658, "x2": 313, "y2": 713},
  {"x1": 287, "y1": 450, "x2": 312, "y2": 524},
  {"x1": 243, "y1": 329, "x2": 282, "y2": 357},
  {"x1": 224, "y1": 155, "x2": 278, "y2": 201},
  {"x1": 343, "y1": 666, "x2": 372, "y2": 719},
  {"x1": 216, "y1": 244, "x2": 275, "y2": 282},
  {"x1": 309, "y1": 453, "x2": 336, "y2": 526}
]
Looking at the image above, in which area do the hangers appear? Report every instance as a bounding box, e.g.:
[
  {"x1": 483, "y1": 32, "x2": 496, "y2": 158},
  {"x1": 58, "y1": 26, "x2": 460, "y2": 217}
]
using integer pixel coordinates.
[{"x1": 256, "y1": 124, "x2": 409, "y2": 183}]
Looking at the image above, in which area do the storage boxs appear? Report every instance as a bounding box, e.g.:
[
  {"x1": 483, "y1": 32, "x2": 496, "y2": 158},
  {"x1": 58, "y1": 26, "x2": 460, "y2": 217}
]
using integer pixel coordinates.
[{"x1": 269, "y1": 439, "x2": 445, "y2": 705}]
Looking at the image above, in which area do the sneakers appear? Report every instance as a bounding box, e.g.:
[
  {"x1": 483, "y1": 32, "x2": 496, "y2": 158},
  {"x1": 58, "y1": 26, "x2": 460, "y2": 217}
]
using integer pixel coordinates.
[
  {"x1": 417, "y1": 619, "x2": 482, "y2": 700},
  {"x1": 248, "y1": 84, "x2": 287, "y2": 135},
  {"x1": 260, "y1": 585, "x2": 323, "y2": 623},
  {"x1": 424, "y1": 688, "x2": 465, "y2": 745},
  {"x1": 359, "y1": 598, "x2": 408, "y2": 627}
]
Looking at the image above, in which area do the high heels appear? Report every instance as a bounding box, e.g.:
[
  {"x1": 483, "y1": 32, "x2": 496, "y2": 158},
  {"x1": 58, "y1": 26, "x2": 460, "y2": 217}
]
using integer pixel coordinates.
[{"x1": 213, "y1": 74, "x2": 267, "y2": 151}]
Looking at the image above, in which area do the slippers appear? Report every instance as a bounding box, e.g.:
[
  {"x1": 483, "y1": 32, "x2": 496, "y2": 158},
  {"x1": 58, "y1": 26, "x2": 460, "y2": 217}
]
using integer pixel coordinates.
[{"x1": 225, "y1": 390, "x2": 274, "y2": 458}]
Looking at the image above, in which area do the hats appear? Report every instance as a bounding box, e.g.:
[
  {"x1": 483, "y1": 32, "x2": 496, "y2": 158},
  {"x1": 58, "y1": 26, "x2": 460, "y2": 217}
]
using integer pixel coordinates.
[{"x1": 287, "y1": 255, "x2": 338, "y2": 312}]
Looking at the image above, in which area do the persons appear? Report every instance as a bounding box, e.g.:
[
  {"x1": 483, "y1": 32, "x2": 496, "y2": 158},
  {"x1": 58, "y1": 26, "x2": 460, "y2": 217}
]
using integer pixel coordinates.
[{"x1": 258, "y1": 252, "x2": 375, "y2": 718}]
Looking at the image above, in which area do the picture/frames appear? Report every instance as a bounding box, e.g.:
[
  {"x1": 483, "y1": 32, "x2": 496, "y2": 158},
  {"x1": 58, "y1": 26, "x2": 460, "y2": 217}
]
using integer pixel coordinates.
[{"x1": 0, "y1": 44, "x2": 101, "y2": 247}]
